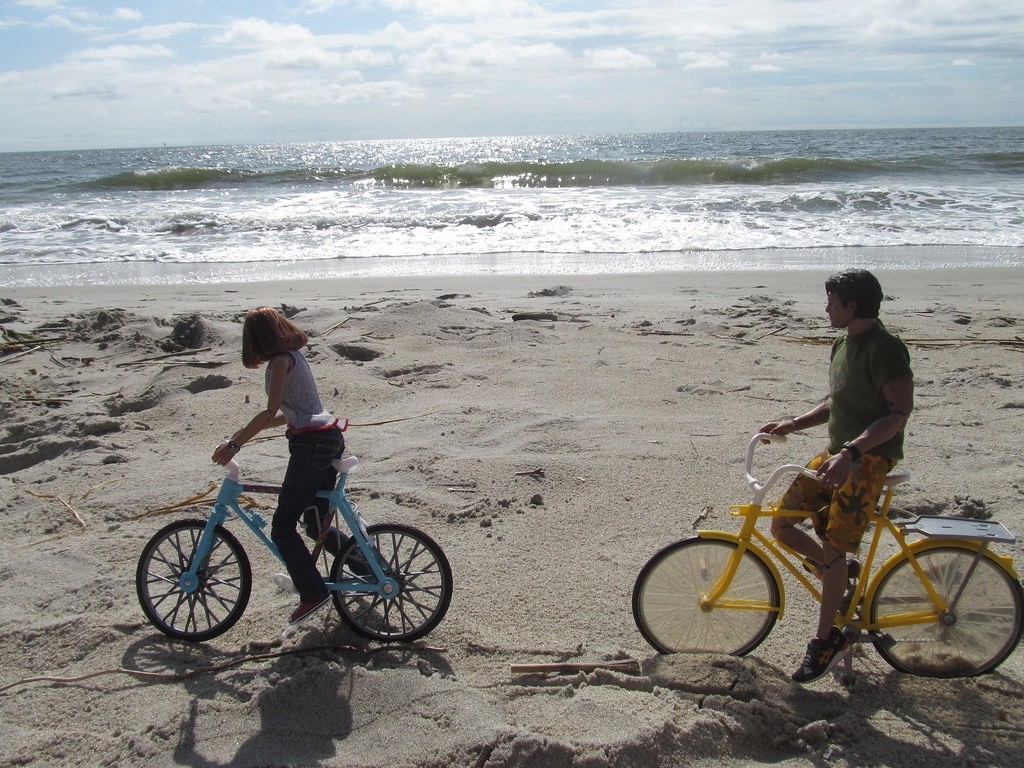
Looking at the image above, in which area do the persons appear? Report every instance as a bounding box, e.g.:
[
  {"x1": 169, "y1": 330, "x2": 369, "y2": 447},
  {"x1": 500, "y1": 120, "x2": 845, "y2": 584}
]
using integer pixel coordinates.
[
  {"x1": 759, "y1": 267, "x2": 913, "y2": 683},
  {"x1": 212, "y1": 306, "x2": 384, "y2": 626}
]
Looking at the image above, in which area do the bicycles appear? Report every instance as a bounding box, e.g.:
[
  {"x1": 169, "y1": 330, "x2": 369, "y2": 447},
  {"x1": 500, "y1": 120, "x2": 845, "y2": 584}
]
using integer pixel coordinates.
[
  {"x1": 631, "y1": 435, "x2": 1024, "y2": 675},
  {"x1": 137, "y1": 444, "x2": 455, "y2": 645}
]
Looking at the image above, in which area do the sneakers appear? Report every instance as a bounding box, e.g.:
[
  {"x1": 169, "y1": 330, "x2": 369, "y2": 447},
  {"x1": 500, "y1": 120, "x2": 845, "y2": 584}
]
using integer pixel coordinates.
[
  {"x1": 790, "y1": 626, "x2": 851, "y2": 684},
  {"x1": 803, "y1": 555, "x2": 862, "y2": 577},
  {"x1": 358, "y1": 568, "x2": 394, "y2": 597},
  {"x1": 288, "y1": 592, "x2": 333, "y2": 626}
]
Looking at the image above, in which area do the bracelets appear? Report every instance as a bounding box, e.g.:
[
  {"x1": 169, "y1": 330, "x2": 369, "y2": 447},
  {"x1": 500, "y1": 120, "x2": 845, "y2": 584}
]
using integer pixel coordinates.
[
  {"x1": 229, "y1": 441, "x2": 240, "y2": 451},
  {"x1": 794, "y1": 418, "x2": 800, "y2": 428}
]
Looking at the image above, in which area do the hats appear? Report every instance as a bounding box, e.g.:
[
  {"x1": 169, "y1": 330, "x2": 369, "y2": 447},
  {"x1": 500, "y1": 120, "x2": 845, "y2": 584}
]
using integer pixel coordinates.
[{"x1": 825, "y1": 268, "x2": 884, "y2": 320}]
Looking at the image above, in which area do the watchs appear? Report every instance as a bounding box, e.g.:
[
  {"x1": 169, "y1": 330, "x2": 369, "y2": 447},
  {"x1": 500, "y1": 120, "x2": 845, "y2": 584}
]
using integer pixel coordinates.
[{"x1": 843, "y1": 441, "x2": 862, "y2": 463}]
